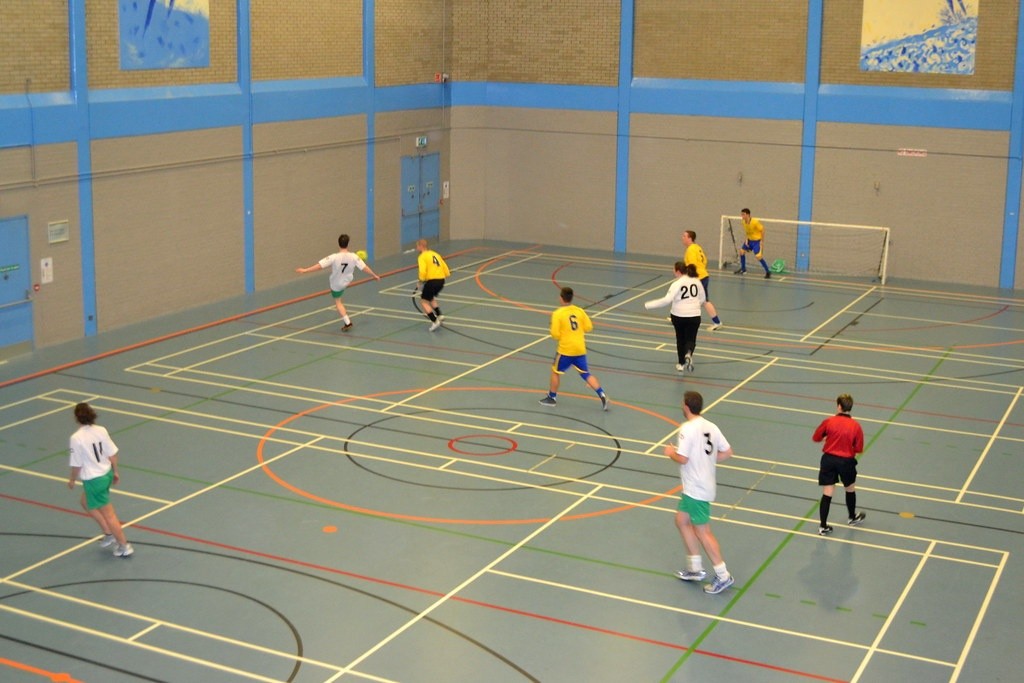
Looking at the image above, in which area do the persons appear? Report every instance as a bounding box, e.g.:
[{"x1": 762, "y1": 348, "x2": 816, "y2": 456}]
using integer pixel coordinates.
[
  {"x1": 65, "y1": 403, "x2": 135, "y2": 556},
  {"x1": 664, "y1": 390, "x2": 735, "y2": 595},
  {"x1": 645, "y1": 262, "x2": 707, "y2": 371},
  {"x1": 415, "y1": 240, "x2": 450, "y2": 331},
  {"x1": 666, "y1": 231, "x2": 722, "y2": 331},
  {"x1": 537, "y1": 286, "x2": 611, "y2": 411},
  {"x1": 812, "y1": 394, "x2": 866, "y2": 536},
  {"x1": 731, "y1": 208, "x2": 772, "y2": 278},
  {"x1": 294, "y1": 234, "x2": 380, "y2": 332}
]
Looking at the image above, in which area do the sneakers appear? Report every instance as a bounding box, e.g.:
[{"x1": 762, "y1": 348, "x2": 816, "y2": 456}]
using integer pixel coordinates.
[
  {"x1": 113, "y1": 543, "x2": 134, "y2": 556},
  {"x1": 342, "y1": 322, "x2": 353, "y2": 332},
  {"x1": 848, "y1": 512, "x2": 865, "y2": 525},
  {"x1": 676, "y1": 364, "x2": 684, "y2": 372},
  {"x1": 708, "y1": 321, "x2": 722, "y2": 331},
  {"x1": 600, "y1": 391, "x2": 607, "y2": 410},
  {"x1": 735, "y1": 268, "x2": 747, "y2": 274},
  {"x1": 685, "y1": 355, "x2": 694, "y2": 373},
  {"x1": 703, "y1": 572, "x2": 734, "y2": 594},
  {"x1": 818, "y1": 526, "x2": 833, "y2": 535},
  {"x1": 539, "y1": 398, "x2": 556, "y2": 406},
  {"x1": 429, "y1": 315, "x2": 444, "y2": 331},
  {"x1": 101, "y1": 533, "x2": 116, "y2": 548},
  {"x1": 764, "y1": 270, "x2": 770, "y2": 278},
  {"x1": 674, "y1": 569, "x2": 706, "y2": 581}
]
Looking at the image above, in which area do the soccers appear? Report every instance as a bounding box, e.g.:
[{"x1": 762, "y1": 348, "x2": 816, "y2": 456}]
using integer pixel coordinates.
[{"x1": 356, "y1": 250, "x2": 369, "y2": 262}]
